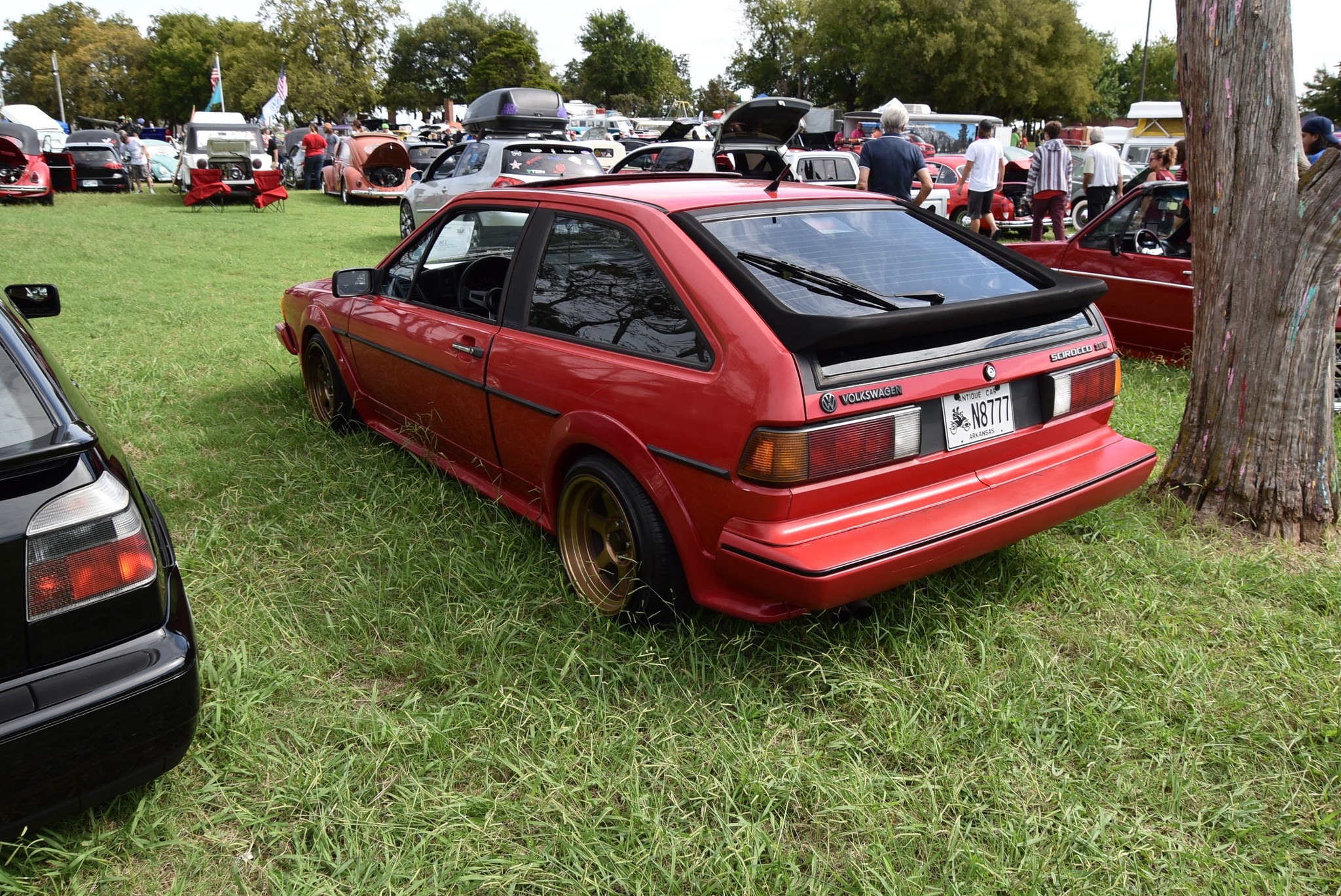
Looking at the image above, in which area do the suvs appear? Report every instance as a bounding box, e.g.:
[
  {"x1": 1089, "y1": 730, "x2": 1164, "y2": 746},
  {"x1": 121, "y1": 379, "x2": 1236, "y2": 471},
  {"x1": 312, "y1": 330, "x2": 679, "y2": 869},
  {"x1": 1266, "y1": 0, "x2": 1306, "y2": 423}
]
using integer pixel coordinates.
[{"x1": 400, "y1": 87, "x2": 606, "y2": 251}]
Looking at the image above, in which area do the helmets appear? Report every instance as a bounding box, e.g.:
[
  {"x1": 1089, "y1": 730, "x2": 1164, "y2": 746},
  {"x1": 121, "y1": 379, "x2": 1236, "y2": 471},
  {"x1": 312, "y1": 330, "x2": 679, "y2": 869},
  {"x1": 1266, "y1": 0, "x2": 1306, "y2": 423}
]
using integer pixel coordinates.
[{"x1": 136, "y1": 118, "x2": 145, "y2": 125}]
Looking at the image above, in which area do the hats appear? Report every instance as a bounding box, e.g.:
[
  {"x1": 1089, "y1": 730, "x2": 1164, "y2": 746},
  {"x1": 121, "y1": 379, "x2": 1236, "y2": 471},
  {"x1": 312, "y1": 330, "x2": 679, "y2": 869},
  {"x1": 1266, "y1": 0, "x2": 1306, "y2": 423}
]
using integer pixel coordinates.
[
  {"x1": 629, "y1": 127, "x2": 635, "y2": 130},
  {"x1": 979, "y1": 119, "x2": 992, "y2": 130},
  {"x1": 382, "y1": 123, "x2": 389, "y2": 128},
  {"x1": 1302, "y1": 116, "x2": 1340, "y2": 144},
  {"x1": 857, "y1": 123, "x2": 863, "y2": 127},
  {"x1": 320, "y1": 122, "x2": 333, "y2": 128},
  {"x1": 569, "y1": 130, "x2": 576, "y2": 135},
  {"x1": 873, "y1": 126, "x2": 880, "y2": 130},
  {"x1": 620, "y1": 129, "x2": 626, "y2": 134}
]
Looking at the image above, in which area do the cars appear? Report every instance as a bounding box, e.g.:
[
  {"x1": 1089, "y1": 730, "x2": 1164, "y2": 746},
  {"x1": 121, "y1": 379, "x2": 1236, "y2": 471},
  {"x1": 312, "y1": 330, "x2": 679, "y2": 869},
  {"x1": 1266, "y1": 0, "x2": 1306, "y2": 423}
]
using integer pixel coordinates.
[
  {"x1": 179, "y1": 112, "x2": 270, "y2": 202},
  {"x1": 274, "y1": 169, "x2": 1159, "y2": 632},
  {"x1": 0, "y1": 122, "x2": 180, "y2": 207},
  {"x1": 995, "y1": 181, "x2": 1340, "y2": 396},
  {"x1": 1, "y1": 282, "x2": 202, "y2": 839},
  {"x1": 273, "y1": 115, "x2": 627, "y2": 204},
  {"x1": 567, "y1": 97, "x2": 1187, "y2": 237}
]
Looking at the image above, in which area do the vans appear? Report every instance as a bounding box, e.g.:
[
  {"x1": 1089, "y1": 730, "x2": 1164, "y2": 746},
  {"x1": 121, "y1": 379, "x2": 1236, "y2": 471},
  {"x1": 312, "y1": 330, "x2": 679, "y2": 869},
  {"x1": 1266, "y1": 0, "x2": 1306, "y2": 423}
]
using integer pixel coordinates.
[
  {"x1": 569, "y1": 114, "x2": 632, "y2": 132},
  {"x1": 635, "y1": 121, "x2": 705, "y2": 138}
]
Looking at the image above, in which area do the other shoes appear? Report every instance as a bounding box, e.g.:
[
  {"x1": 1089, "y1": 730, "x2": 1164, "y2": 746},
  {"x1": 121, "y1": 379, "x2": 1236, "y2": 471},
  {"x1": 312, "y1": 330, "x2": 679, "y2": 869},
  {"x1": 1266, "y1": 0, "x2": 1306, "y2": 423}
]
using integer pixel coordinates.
[
  {"x1": 988, "y1": 229, "x2": 1002, "y2": 241},
  {"x1": 149, "y1": 189, "x2": 155, "y2": 195},
  {"x1": 136, "y1": 190, "x2": 143, "y2": 194}
]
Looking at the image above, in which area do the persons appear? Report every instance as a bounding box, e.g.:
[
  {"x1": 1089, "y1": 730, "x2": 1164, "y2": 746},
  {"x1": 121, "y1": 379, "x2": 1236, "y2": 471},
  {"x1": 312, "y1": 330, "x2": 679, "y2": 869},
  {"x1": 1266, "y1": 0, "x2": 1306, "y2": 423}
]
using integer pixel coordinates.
[
  {"x1": 432, "y1": 133, "x2": 438, "y2": 142},
  {"x1": 301, "y1": 123, "x2": 328, "y2": 190},
  {"x1": 119, "y1": 130, "x2": 156, "y2": 195},
  {"x1": 569, "y1": 130, "x2": 579, "y2": 141},
  {"x1": 118, "y1": 114, "x2": 156, "y2": 128},
  {"x1": 334, "y1": 130, "x2": 344, "y2": 137},
  {"x1": 1011, "y1": 126, "x2": 1042, "y2": 150},
  {"x1": 628, "y1": 128, "x2": 639, "y2": 138},
  {"x1": 351, "y1": 120, "x2": 364, "y2": 134},
  {"x1": 957, "y1": 120, "x2": 1005, "y2": 242},
  {"x1": 619, "y1": 130, "x2": 627, "y2": 140},
  {"x1": 1300, "y1": 116, "x2": 1341, "y2": 164},
  {"x1": 851, "y1": 123, "x2": 866, "y2": 138},
  {"x1": 261, "y1": 123, "x2": 289, "y2": 169},
  {"x1": 1135, "y1": 140, "x2": 1190, "y2": 238},
  {"x1": 604, "y1": 133, "x2": 614, "y2": 141},
  {"x1": 381, "y1": 123, "x2": 394, "y2": 134},
  {"x1": 871, "y1": 126, "x2": 882, "y2": 138},
  {"x1": 1082, "y1": 126, "x2": 1124, "y2": 223},
  {"x1": 320, "y1": 122, "x2": 340, "y2": 183},
  {"x1": 453, "y1": 128, "x2": 463, "y2": 143},
  {"x1": 1026, "y1": 121, "x2": 1073, "y2": 242},
  {"x1": 856, "y1": 103, "x2": 933, "y2": 206},
  {"x1": 442, "y1": 128, "x2": 451, "y2": 147}
]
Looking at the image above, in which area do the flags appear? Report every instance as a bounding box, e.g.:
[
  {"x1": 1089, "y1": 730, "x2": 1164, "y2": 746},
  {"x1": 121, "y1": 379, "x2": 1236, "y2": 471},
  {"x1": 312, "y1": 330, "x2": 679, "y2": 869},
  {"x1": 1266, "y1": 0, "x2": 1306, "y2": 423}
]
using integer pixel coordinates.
[
  {"x1": 277, "y1": 59, "x2": 289, "y2": 101},
  {"x1": 204, "y1": 59, "x2": 222, "y2": 112}
]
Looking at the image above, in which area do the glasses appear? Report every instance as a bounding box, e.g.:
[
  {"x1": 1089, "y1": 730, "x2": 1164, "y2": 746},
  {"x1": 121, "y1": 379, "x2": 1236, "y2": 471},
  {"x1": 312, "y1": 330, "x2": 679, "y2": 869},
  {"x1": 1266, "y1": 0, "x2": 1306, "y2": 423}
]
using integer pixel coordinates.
[
  {"x1": 619, "y1": 133, "x2": 622, "y2": 135},
  {"x1": 1149, "y1": 157, "x2": 1157, "y2": 161}
]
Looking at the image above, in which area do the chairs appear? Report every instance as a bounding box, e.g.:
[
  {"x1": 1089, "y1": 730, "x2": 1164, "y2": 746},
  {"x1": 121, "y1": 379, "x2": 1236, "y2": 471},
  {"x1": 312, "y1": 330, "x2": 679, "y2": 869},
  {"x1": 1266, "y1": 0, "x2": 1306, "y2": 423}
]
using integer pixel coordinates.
[
  {"x1": 183, "y1": 167, "x2": 231, "y2": 213},
  {"x1": 245, "y1": 169, "x2": 289, "y2": 213}
]
